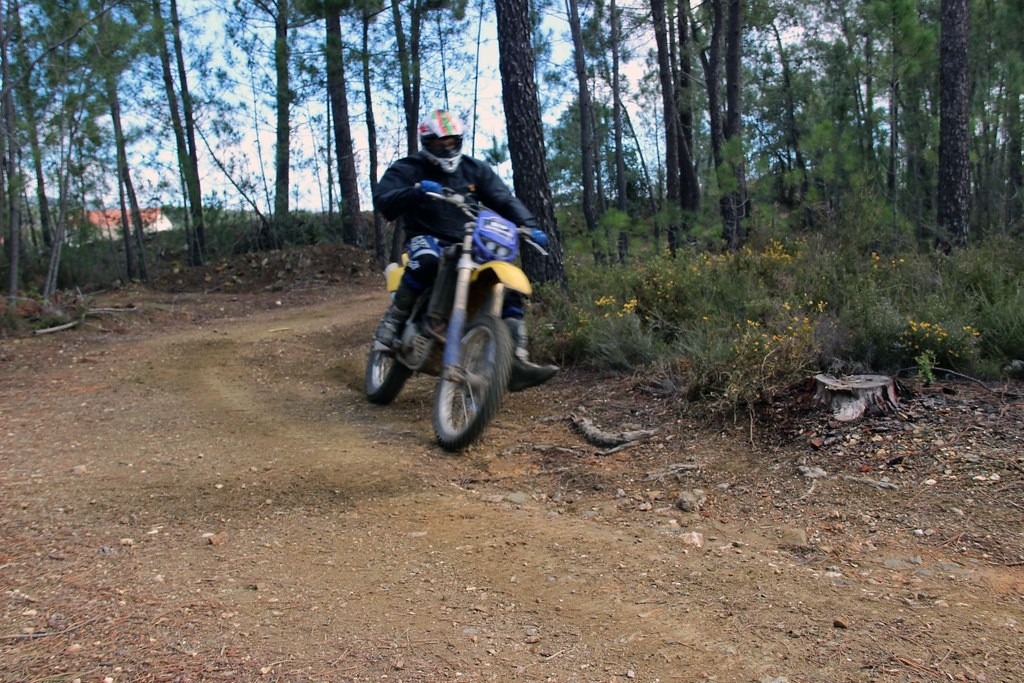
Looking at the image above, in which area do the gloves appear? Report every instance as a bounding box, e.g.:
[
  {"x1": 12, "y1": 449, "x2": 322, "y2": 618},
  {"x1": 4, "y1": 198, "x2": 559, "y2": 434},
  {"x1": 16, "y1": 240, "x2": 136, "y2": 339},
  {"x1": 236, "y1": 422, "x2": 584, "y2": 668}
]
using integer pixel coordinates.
[
  {"x1": 532, "y1": 228, "x2": 549, "y2": 245},
  {"x1": 414, "y1": 181, "x2": 442, "y2": 200}
]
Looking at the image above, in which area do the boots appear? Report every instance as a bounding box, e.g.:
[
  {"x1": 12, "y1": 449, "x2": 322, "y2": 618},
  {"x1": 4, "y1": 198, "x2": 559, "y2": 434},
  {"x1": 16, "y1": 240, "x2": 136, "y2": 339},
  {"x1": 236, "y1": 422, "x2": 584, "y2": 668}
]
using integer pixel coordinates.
[
  {"x1": 504, "y1": 318, "x2": 561, "y2": 393},
  {"x1": 376, "y1": 304, "x2": 410, "y2": 345}
]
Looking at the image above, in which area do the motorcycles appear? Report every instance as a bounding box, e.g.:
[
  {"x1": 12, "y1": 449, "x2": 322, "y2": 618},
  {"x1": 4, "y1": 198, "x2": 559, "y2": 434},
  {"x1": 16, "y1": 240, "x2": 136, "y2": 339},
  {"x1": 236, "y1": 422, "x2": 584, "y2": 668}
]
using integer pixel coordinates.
[{"x1": 365, "y1": 183, "x2": 546, "y2": 450}]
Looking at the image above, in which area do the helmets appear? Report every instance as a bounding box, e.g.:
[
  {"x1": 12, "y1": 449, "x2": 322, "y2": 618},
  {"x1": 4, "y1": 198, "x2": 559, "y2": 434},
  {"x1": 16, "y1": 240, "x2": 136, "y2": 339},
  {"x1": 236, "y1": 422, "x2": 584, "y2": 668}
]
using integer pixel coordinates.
[{"x1": 421, "y1": 109, "x2": 465, "y2": 173}]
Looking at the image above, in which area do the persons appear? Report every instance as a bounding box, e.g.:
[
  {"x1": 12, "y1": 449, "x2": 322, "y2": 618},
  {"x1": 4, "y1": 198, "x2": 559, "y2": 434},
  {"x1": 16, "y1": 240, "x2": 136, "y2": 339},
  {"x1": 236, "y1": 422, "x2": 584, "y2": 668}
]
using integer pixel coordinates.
[{"x1": 372, "y1": 107, "x2": 559, "y2": 391}]
